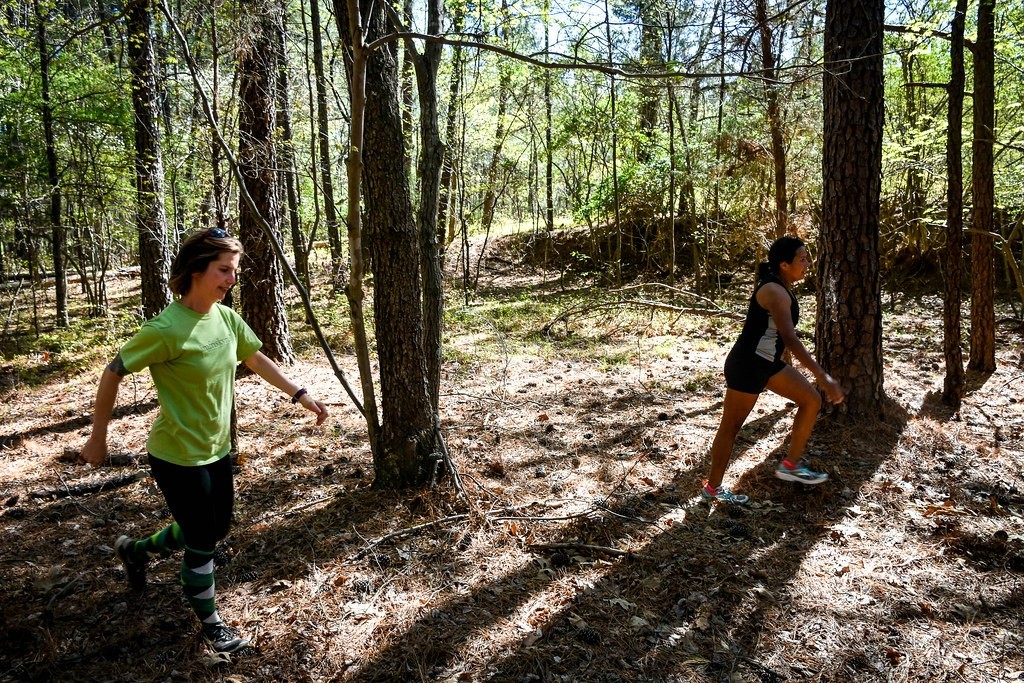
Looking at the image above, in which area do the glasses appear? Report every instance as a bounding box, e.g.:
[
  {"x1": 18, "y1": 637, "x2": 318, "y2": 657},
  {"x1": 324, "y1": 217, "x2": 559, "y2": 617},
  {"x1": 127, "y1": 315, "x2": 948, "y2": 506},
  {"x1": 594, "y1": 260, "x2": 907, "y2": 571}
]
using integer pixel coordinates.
[{"x1": 196, "y1": 227, "x2": 232, "y2": 246}]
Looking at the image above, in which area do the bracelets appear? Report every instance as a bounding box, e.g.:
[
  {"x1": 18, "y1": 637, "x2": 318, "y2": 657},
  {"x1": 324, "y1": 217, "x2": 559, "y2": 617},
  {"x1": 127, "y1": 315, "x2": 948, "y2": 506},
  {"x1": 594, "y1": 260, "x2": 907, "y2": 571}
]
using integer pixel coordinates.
[{"x1": 292, "y1": 389, "x2": 307, "y2": 404}]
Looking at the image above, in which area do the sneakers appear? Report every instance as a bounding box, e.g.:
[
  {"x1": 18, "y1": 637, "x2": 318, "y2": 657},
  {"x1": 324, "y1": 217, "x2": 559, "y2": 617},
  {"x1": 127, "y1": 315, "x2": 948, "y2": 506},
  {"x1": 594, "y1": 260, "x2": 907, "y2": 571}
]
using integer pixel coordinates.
[
  {"x1": 115, "y1": 535, "x2": 148, "y2": 592},
  {"x1": 199, "y1": 621, "x2": 242, "y2": 651},
  {"x1": 773, "y1": 459, "x2": 829, "y2": 484},
  {"x1": 700, "y1": 483, "x2": 748, "y2": 505}
]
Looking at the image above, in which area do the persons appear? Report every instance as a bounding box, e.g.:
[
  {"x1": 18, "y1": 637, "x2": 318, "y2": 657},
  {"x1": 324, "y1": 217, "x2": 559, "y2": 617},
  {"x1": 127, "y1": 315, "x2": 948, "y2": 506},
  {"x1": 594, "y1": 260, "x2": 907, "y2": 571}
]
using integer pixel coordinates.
[
  {"x1": 703, "y1": 237, "x2": 844, "y2": 503},
  {"x1": 81, "y1": 228, "x2": 329, "y2": 654}
]
